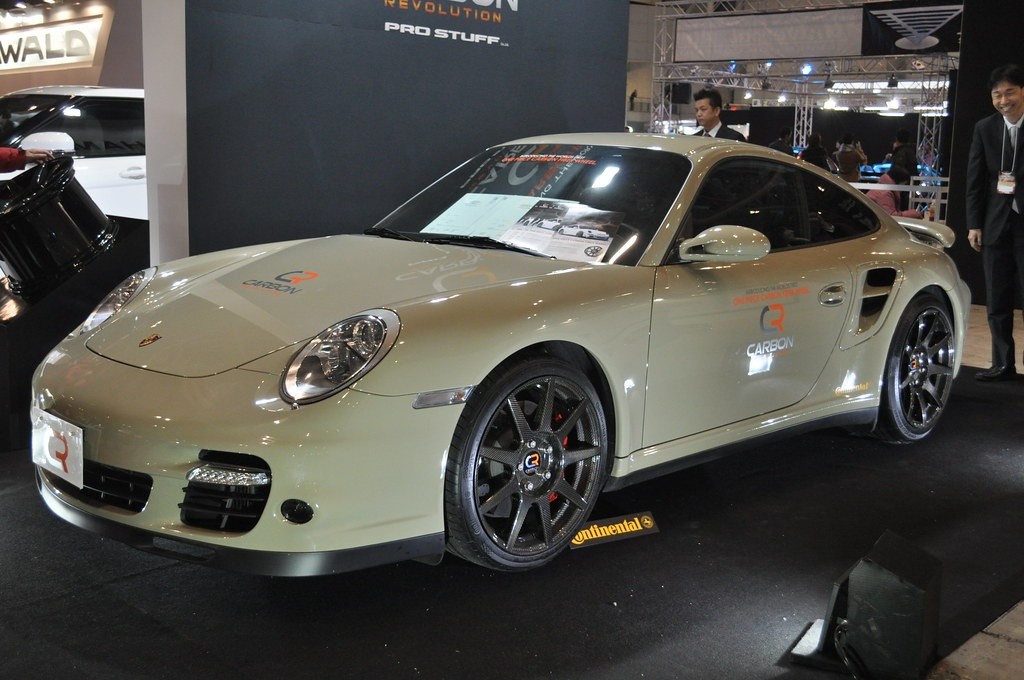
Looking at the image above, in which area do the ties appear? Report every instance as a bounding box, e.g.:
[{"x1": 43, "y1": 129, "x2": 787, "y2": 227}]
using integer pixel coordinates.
[
  {"x1": 704, "y1": 133, "x2": 710, "y2": 136},
  {"x1": 1010, "y1": 126, "x2": 1017, "y2": 152}
]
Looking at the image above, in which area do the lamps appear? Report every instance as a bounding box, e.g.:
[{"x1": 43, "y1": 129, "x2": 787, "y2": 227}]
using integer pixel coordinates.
[
  {"x1": 761, "y1": 77, "x2": 771, "y2": 90},
  {"x1": 824, "y1": 74, "x2": 835, "y2": 89},
  {"x1": 788, "y1": 527, "x2": 944, "y2": 680},
  {"x1": 887, "y1": 73, "x2": 900, "y2": 89}
]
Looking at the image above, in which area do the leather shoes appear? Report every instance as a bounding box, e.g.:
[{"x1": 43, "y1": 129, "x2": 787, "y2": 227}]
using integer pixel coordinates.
[{"x1": 976, "y1": 365, "x2": 1016, "y2": 381}]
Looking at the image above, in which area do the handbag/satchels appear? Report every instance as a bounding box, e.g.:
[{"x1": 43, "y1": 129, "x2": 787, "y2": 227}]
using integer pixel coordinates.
[{"x1": 825, "y1": 155, "x2": 840, "y2": 173}]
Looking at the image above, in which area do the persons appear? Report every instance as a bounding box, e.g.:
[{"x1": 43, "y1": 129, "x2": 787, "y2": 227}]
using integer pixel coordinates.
[
  {"x1": 965, "y1": 65, "x2": 1024, "y2": 381},
  {"x1": 767, "y1": 126, "x2": 937, "y2": 220},
  {"x1": 689, "y1": 88, "x2": 748, "y2": 143},
  {"x1": 0, "y1": 144, "x2": 56, "y2": 173}
]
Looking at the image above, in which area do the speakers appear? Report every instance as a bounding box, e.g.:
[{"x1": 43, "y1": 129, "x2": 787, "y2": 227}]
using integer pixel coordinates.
[{"x1": 665, "y1": 83, "x2": 692, "y2": 104}]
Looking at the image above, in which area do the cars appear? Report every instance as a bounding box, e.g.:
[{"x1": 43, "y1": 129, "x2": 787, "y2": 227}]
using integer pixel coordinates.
[
  {"x1": 0, "y1": 85, "x2": 146, "y2": 223},
  {"x1": 536, "y1": 216, "x2": 609, "y2": 240}
]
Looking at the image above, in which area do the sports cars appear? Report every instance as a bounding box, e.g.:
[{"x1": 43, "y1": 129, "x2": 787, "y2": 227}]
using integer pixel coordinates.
[{"x1": 30, "y1": 133, "x2": 971, "y2": 574}]
